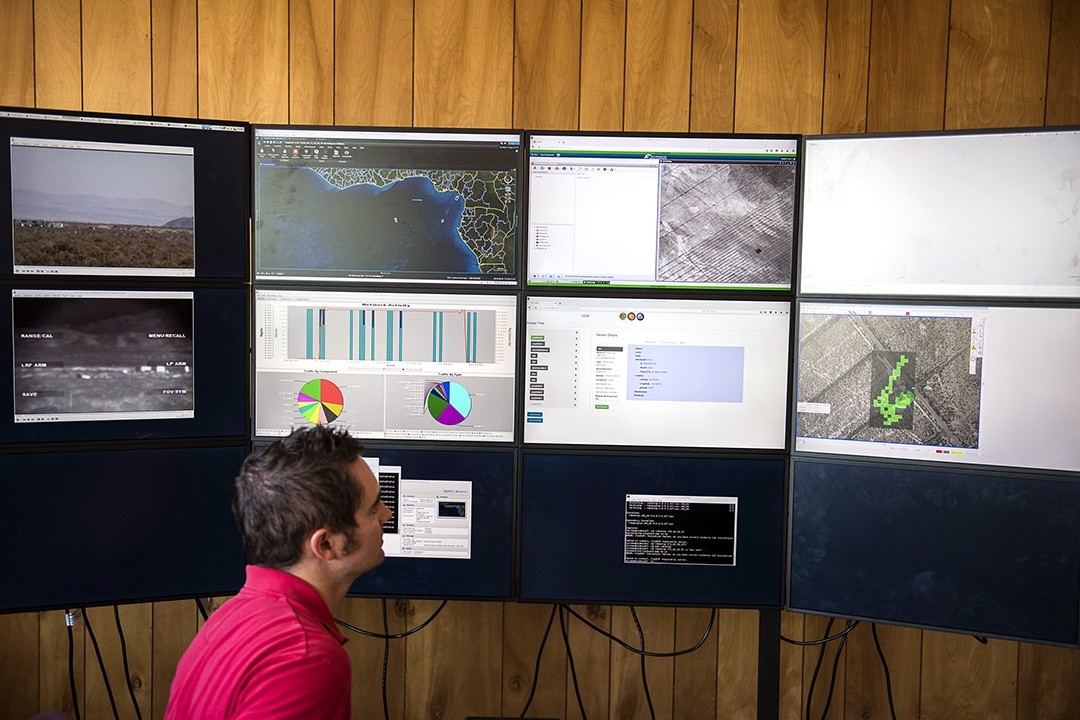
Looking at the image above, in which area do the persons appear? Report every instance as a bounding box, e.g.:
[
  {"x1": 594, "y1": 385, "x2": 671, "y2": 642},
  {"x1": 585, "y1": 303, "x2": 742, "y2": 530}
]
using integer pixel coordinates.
[{"x1": 162, "y1": 424, "x2": 394, "y2": 720}]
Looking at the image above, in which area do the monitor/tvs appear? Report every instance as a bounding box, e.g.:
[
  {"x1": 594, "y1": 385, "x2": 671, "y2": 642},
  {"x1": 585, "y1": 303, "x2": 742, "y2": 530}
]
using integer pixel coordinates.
[{"x1": 1, "y1": 107, "x2": 1080, "y2": 653}]
